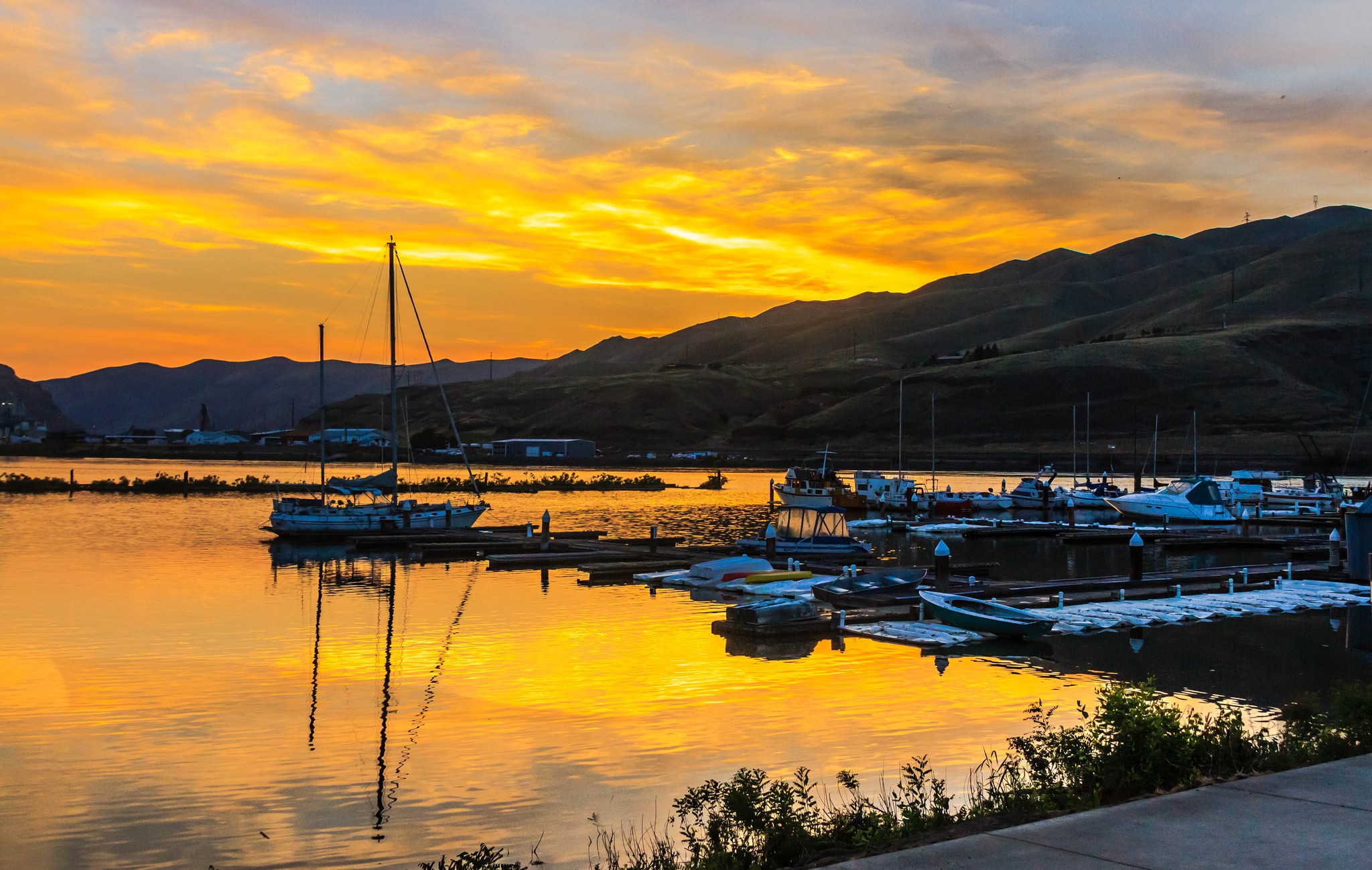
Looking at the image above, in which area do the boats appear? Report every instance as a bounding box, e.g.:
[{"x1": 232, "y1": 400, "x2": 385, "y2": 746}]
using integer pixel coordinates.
[
  {"x1": 772, "y1": 441, "x2": 867, "y2": 507},
  {"x1": 919, "y1": 590, "x2": 1060, "y2": 635},
  {"x1": 999, "y1": 391, "x2": 1372, "y2": 521},
  {"x1": 811, "y1": 569, "x2": 927, "y2": 603},
  {"x1": 854, "y1": 377, "x2": 1012, "y2": 510},
  {"x1": 736, "y1": 504, "x2": 871, "y2": 554}
]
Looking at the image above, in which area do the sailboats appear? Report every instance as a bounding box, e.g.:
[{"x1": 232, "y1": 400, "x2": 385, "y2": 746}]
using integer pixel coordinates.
[{"x1": 258, "y1": 234, "x2": 490, "y2": 537}]
